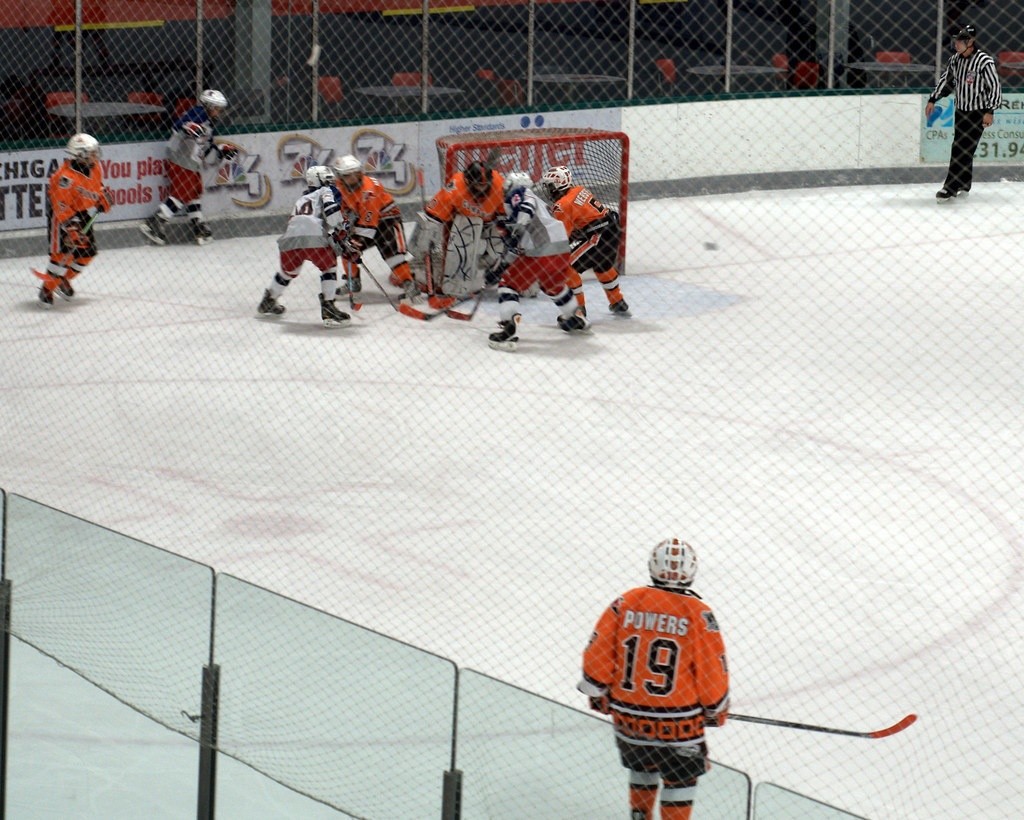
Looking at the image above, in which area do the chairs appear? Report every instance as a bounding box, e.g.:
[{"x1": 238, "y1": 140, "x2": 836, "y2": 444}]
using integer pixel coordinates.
[
  {"x1": 317, "y1": 77, "x2": 346, "y2": 119},
  {"x1": 495, "y1": 78, "x2": 524, "y2": 107},
  {"x1": 392, "y1": 73, "x2": 432, "y2": 86},
  {"x1": 45, "y1": 93, "x2": 90, "y2": 136},
  {"x1": 656, "y1": 59, "x2": 675, "y2": 83},
  {"x1": 773, "y1": 55, "x2": 788, "y2": 82},
  {"x1": 476, "y1": 68, "x2": 496, "y2": 80},
  {"x1": 174, "y1": 96, "x2": 196, "y2": 121},
  {"x1": 128, "y1": 92, "x2": 163, "y2": 123},
  {"x1": 876, "y1": 51, "x2": 911, "y2": 65},
  {"x1": 794, "y1": 62, "x2": 819, "y2": 89},
  {"x1": 997, "y1": 51, "x2": 1024, "y2": 76}
]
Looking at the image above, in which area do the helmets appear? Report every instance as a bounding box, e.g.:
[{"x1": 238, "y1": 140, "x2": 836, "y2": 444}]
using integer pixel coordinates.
[
  {"x1": 501, "y1": 172, "x2": 536, "y2": 197},
  {"x1": 952, "y1": 24, "x2": 977, "y2": 41},
  {"x1": 198, "y1": 89, "x2": 228, "y2": 108},
  {"x1": 63, "y1": 132, "x2": 100, "y2": 159},
  {"x1": 646, "y1": 536, "x2": 698, "y2": 590},
  {"x1": 541, "y1": 165, "x2": 572, "y2": 194},
  {"x1": 333, "y1": 154, "x2": 363, "y2": 175},
  {"x1": 305, "y1": 165, "x2": 336, "y2": 191},
  {"x1": 463, "y1": 160, "x2": 493, "y2": 185}
]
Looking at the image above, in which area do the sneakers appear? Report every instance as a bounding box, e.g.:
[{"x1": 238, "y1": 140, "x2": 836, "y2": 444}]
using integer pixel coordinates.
[
  {"x1": 935, "y1": 187, "x2": 970, "y2": 203},
  {"x1": 53, "y1": 277, "x2": 76, "y2": 302},
  {"x1": 138, "y1": 217, "x2": 168, "y2": 246},
  {"x1": 335, "y1": 274, "x2": 362, "y2": 301},
  {"x1": 256, "y1": 288, "x2": 286, "y2": 316},
  {"x1": 608, "y1": 298, "x2": 629, "y2": 316},
  {"x1": 556, "y1": 314, "x2": 592, "y2": 336},
  {"x1": 194, "y1": 225, "x2": 214, "y2": 246},
  {"x1": 317, "y1": 293, "x2": 353, "y2": 329},
  {"x1": 396, "y1": 288, "x2": 424, "y2": 308},
  {"x1": 486, "y1": 320, "x2": 519, "y2": 353},
  {"x1": 35, "y1": 286, "x2": 56, "y2": 310}
]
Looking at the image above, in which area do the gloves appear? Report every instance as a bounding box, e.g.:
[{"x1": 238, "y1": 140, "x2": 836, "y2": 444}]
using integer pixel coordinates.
[
  {"x1": 216, "y1": 143, "x2": 238, "y2": 160},
  {"x1": 94, "y1": 186, "x2": 116, "y2": 213},
  {"x1": 181, "y1": 120, "x2": 207, "y2": 141},
  {"x1": 63, "y1": 222, "x2": 92, "y2": 249},
  {"x1": 588, "y1": 695, "x2": 611, "y2": 715},
  {"x1": 703, "y1": 708, "x2": 729, "y2": 728}
]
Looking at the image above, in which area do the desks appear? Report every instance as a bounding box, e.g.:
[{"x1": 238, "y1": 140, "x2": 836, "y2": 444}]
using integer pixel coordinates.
[
  {"x1": 48, "y1": 102, "x2": 166, "y2": 134},
  {"x1": 1000, "y1": 62, "x2": 1024, "y2": 72},
  {"x1": 354, "y1": 86, "x2": 466, "y2": 115},
  {"x1": 521, "y1": 74, "x2": 627, "y2": 104},
  {"x1": 685, "y1": 64, "x2": 789, "y2": 92},
  {"x1": 843, "y1": 62, "x2": 948, "y2": 87}
]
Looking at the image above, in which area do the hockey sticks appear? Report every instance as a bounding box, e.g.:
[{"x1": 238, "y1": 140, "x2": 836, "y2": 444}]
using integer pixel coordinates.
[
  {"x1": 398, "y1": 281, "x2": 500, "y2": 322},
  {"x1": 417, "y1": 169, "x2": 454, "y2": 309},
  {"x1": 347, "y1": 261, "x2": 363, "y2": 312},
  {"x1": 359, "y1": 259, "x2": 414, "y2": 313},
  {"x1": 445, "y1": 247, "x2": 508, "y2": 321},
  {"x1": 28, "y1": 207, "x2": 103, "y2": 282},
  {"x1": 724, "y1": 711, "x2": 918, "y2": 739}
]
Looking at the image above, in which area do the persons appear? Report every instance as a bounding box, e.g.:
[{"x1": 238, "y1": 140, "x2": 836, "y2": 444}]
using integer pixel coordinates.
[
  {"x1": 335, "y1": 155, "x2": 422, "y2": 305},
  {"x1": 577, "y1": 539, "x2": 731, "y2": 820},
  {"x1": 925, "y1": 24, "x2": 1001, "y2": 203},
  {"x1": 37, "y1": 133, "x2": 114, "y2": 309},
  {"x1": 388, "y1": 161, "x2": 538, "y2": 298},
  {"x1": 256, "y1": 165, "x2": 352, "y2": 328},
  {"x1": 542, "y1": 166, "x2": 632, "y2": 317},
  {"x1": 488, "y1": 172, "x2": 593, "y2": 351},
  {"x1": 139, "y1": 90, "x2": 238, "y2": 244}
]
